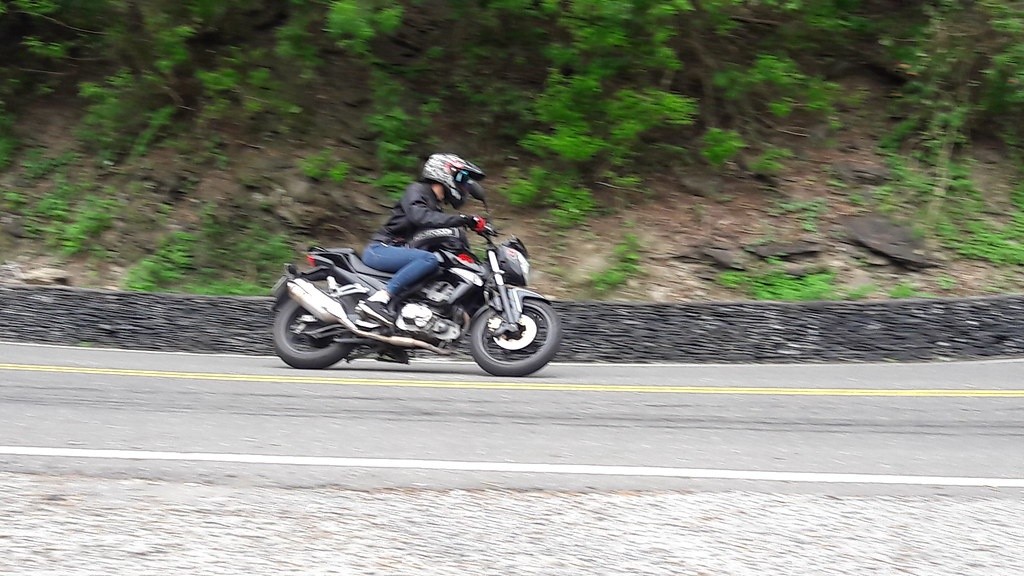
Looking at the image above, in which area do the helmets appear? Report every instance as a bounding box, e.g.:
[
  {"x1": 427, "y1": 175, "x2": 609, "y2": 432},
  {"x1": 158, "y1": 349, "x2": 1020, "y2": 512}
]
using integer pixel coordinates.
[{"x1": 422, "y1": 152, "x2": 486, "y2": 210}]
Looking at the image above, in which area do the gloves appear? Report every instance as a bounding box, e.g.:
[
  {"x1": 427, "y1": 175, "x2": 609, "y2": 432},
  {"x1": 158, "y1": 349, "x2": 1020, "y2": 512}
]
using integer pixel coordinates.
[
  {"x1": 469, "y1": 212, "x2": 485, "y2": 232},
  {"x1": 458, "y1": 251, "x2": 475, "y2": 263}
]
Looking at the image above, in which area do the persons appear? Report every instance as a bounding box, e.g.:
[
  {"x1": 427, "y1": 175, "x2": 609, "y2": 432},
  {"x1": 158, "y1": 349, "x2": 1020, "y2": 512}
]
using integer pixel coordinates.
[{"x1": 355, "y1": 153, "x2": 487, "y2": 325}]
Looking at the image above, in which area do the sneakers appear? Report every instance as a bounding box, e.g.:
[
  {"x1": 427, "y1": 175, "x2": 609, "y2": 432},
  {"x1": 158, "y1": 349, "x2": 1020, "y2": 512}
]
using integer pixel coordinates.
[{"x1": 358, "y1": 298, "x2": 394, "y2": 326}]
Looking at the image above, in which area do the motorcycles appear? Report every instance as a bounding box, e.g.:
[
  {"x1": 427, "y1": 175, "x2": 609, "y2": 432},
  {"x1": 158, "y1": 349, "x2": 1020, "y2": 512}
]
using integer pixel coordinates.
[{"x1": 268, "y1": 177, "x2": 563, "y2": 378}]
